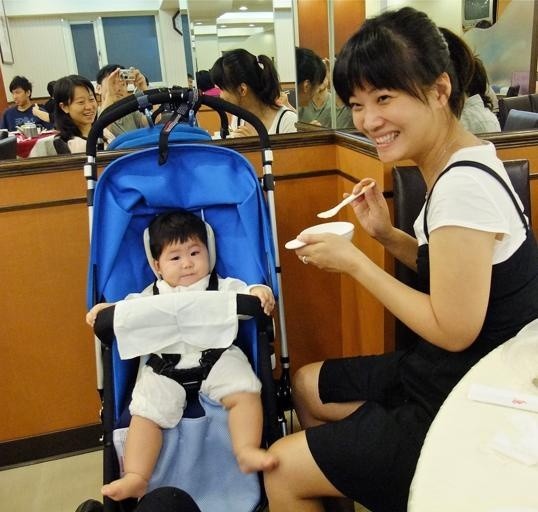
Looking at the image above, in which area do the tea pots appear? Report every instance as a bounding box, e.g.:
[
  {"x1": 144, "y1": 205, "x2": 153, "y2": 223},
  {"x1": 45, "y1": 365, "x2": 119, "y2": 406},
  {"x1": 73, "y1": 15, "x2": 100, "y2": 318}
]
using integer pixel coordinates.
[{"x1": 15, "y1": 120, "x2": 39, "y2": 139}]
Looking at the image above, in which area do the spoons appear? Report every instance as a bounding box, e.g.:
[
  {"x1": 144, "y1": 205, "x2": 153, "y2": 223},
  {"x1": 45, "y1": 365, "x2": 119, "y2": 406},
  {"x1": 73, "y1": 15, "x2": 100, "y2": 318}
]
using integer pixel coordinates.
[{"x1": 315, "y1": 182, "x2": 375, "y2": 219}]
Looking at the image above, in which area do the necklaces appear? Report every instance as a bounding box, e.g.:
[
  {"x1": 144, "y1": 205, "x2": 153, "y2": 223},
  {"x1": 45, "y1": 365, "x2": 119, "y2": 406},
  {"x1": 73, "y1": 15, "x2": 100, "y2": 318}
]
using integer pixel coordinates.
[{"x1": 412, "y1": 129, "x2": 469, "y2": 201}]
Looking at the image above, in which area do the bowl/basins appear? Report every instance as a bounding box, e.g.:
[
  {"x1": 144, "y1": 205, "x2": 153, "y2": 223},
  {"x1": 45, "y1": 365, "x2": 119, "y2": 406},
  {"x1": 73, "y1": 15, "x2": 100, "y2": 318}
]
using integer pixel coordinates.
[{"x1": 284, "y1": 221, "x2": 356, "y2": 249}]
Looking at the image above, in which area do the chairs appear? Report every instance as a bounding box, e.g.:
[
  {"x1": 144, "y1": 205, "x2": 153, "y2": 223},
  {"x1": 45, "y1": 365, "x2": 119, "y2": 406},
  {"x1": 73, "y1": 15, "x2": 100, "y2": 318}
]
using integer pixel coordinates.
[
  {"x1": 390, "y1": 159, "x2": 531, "y2": 356},
  {"x1": 483, "y1": 84, "x2": 538, "y2": 132},
  {"x1": 0, "y1": 129, "x2": 17, "y2": 160}
]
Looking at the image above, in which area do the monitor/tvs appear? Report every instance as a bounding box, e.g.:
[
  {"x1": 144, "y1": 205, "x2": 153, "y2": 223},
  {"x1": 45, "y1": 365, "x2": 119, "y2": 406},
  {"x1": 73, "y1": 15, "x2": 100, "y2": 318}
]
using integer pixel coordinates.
[{"x1": 460, "y1": 0, "x2": 497, "y2": 30}]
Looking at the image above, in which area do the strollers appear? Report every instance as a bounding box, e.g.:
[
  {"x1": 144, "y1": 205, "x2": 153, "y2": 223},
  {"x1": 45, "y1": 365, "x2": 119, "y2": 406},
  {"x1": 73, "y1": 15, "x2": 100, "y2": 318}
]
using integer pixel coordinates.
[{"x1": 76, "y1": 85, "x2": 295, "y2": 511}]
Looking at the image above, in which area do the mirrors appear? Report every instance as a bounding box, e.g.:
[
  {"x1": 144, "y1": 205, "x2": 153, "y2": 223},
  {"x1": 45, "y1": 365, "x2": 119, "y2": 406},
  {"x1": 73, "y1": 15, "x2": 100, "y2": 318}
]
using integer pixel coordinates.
[
  {"x1": 330, "y1": 0, "x2": 538, "y2": 143},
  {"x1": 0, "y1": 0, "x2": 336, "y2": 178}
]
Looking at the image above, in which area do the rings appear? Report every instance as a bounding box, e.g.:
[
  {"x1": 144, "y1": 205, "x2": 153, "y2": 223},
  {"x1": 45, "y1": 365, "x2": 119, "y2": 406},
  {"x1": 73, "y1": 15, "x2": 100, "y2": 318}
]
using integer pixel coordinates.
[{"x1": 300, "y1": 254, "x2": 310, "y2": 266}]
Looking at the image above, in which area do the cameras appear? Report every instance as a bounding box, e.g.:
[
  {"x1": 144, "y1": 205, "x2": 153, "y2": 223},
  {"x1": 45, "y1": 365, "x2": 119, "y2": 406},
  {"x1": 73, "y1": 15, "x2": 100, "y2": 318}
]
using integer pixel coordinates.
[{"x1": 118, "y1": 69, "x2": 135, "y2": 81}]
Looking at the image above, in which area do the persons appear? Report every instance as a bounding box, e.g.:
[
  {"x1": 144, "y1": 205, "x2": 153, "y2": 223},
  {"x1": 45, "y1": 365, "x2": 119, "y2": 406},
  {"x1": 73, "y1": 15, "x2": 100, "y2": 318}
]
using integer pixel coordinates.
[
  {"x1": 459, "y1": 56, "x2": 501, "y2": 134},
  {"x1": 262, "y1": 6, "x2": 538, "y2": 507},
  {"x1": 84, "y1": 208, "x2": 279, "y2": 501},
  {"x1": 2, "y1": 48, "x2": 297, "y2": 159},
  {"x1": 295, "y1": 47, "x2": 355, "y2": 128}
]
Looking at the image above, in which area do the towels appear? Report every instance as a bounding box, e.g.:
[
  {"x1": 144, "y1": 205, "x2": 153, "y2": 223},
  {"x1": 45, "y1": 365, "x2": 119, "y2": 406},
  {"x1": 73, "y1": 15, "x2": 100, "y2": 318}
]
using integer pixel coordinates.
[{"x1": 112, "y1": 291, "x2": 239, "y2": 360}]
